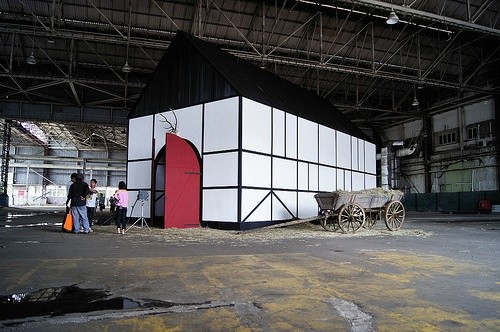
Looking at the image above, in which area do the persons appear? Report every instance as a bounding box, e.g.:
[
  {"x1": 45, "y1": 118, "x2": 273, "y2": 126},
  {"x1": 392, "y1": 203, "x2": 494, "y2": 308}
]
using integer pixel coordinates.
[
  {"x1": 66, "y1": 172, "x2": 95, "y2": 234},
  {"x1": 85, "y1": 178, "x2": 99, "y2": 229},
  {"x1": 113, "y1": 181, "x2": 130, "y2": 234},
  {"x1": 62, "y1": 172, "x2": 80, "y2": 231},
  {"x1": 95, "y1": 189, "x2": 120, "y2": 213}
]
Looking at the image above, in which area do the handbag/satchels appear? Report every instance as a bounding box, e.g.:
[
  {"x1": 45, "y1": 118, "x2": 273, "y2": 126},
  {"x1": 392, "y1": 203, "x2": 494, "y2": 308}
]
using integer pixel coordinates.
[{"x1": 63, "y1": 208, "x2": 74, "y2": 231}]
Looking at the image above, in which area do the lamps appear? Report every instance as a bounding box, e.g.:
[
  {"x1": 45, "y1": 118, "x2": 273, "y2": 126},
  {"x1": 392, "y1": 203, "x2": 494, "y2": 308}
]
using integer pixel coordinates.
[
  {"x1": 122, "y1": 61, "x2": 131, "y2": 73},
  {"x1": 385, "y1": 0, "x2": 399, "y2": 25},
  {"x1": 26, "y1": 51, "x2": 37, "y2": 65},
  {"x1": 412, "y1": 81, "x2": 419, "y2": 106}
]
